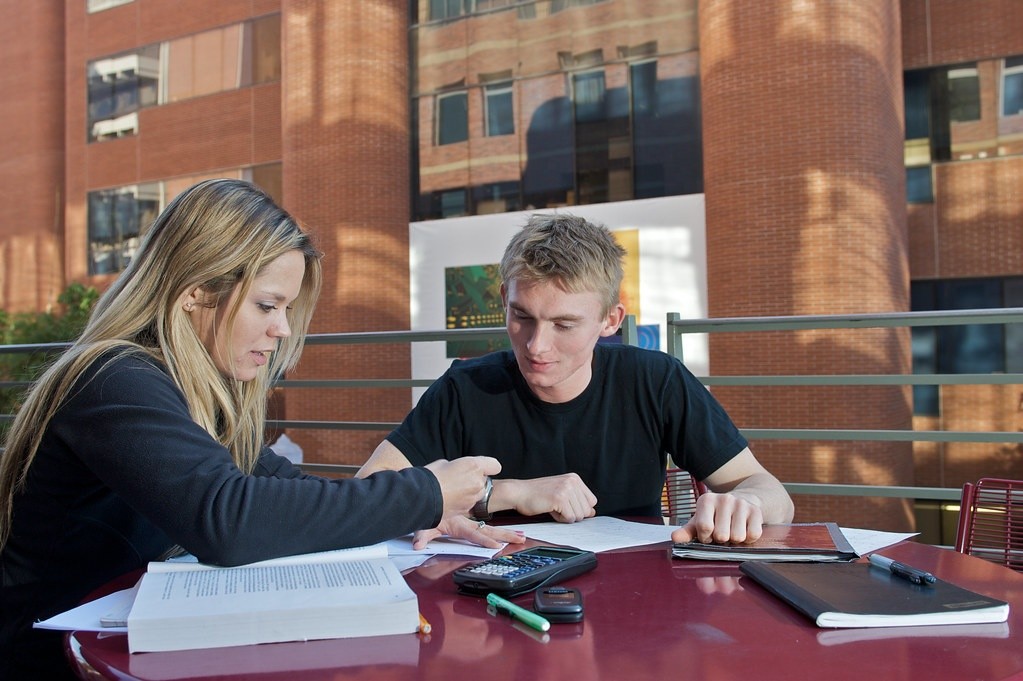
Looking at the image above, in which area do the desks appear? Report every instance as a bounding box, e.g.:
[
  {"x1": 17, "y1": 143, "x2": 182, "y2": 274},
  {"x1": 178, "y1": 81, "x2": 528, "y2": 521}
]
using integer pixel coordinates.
[{"x1": 69, "y1": 542, "x2": 1023, "y2": 681}]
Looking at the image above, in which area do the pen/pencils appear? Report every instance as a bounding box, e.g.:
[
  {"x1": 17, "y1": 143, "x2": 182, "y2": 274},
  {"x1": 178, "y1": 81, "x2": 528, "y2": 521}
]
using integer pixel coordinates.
[
  {"x1": 487, "y1": 593, "x2": 551, "y2": 634},
  {"x1": 866, "y1": 552, "x2": 938, "y2": 587},
  {"x1": 419, "y1": 612, "x2": 431, "y2": 634}
]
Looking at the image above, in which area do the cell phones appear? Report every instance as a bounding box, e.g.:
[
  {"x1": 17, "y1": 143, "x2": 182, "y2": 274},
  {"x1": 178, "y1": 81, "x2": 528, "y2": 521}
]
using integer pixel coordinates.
[{"x1": 534, "y1": 586, "x2": 584, "y2": 623}]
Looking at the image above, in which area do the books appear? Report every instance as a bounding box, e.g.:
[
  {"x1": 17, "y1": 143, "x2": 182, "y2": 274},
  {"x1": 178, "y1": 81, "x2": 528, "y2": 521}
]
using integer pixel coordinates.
[
  {"x1": 737, "y1": 560, "x2": 1012, "y2": 628},
  {"x1": 126, "y1": 544, "x2": 421, "y2": 655},
  {"x1": 671, "y1": 522, "x2": 862, "y2": 563},
  {"x1": 99, "y1": 572, "x2": 146, "y2": 627}
]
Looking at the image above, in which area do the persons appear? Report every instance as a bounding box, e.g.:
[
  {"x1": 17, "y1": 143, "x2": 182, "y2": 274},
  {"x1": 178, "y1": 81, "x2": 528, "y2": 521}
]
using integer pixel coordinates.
[
  {"x1": 0, "y1": 177, "x2": 527, "y2": 681},
  {"x1": 355, "y1": 210, "x2": 795, "y2": 545}
]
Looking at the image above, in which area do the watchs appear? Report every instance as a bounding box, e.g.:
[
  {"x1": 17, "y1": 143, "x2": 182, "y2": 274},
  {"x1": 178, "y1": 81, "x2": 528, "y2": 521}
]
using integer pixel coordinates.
[{"x1": 471, "y1": 476, "x2": 494, "y2": 522}]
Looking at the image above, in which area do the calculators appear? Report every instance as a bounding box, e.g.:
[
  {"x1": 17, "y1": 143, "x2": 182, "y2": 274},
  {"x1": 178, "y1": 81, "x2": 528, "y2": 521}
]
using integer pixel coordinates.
[{"x1": 452, "y1": 545, "x2": 597, "y2": 600}]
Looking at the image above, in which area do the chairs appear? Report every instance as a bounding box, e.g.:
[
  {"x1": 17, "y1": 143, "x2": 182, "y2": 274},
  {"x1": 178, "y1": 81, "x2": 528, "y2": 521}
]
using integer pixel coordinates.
[{"x1": 953, "y1": 478, "x2": 1023, "y2": 574}]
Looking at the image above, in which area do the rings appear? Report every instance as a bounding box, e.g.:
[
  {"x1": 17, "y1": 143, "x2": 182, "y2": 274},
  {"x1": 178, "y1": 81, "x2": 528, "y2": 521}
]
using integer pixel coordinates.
[{"x1": 476, "y1": 521, "x2": 485, "y2": 530}]
